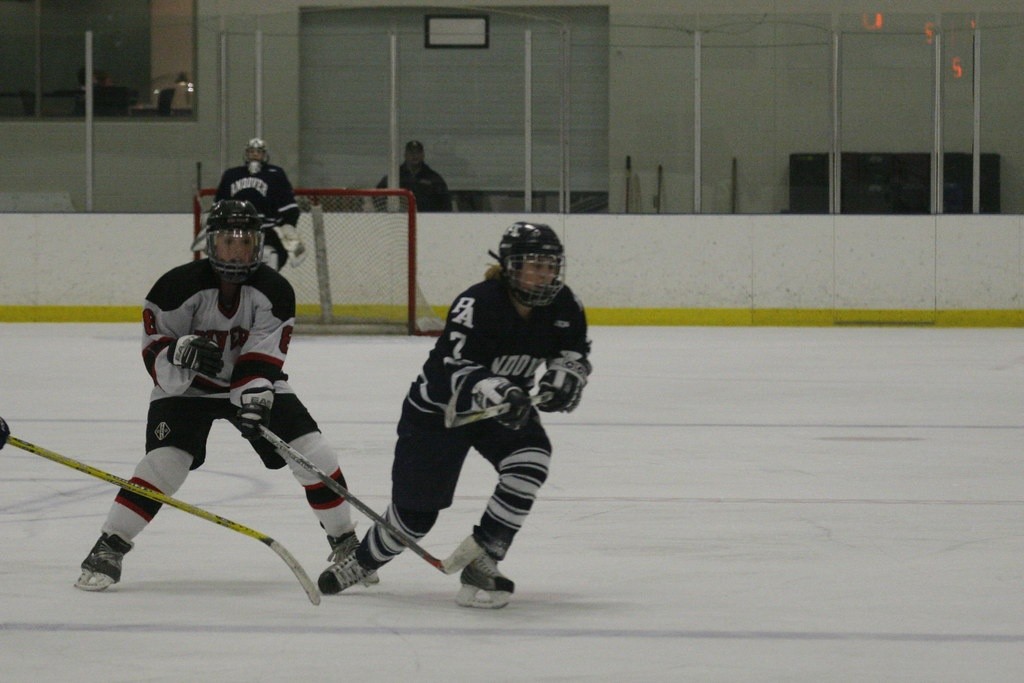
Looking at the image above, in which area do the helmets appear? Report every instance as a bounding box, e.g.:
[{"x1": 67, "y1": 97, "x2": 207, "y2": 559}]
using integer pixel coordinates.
[
  {"x1": 500, "y1": 221, "x2": 565, "y2": 308},
  {"x1": 242, "y1": 139, "x2": 269, "y2": 174},
  {"x1": 206, "y1": 199, "x2": 265, "y2": 284}
]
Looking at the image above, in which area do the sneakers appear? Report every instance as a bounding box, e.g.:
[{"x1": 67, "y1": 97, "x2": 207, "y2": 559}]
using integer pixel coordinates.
[
  {"x1": 74, "y1": 532, "x2": 134, "y2": 591},
  {"x1": 319, "y1": 521, "x2": 379, "y2": 583},
  {"x1": 457, "y1": 525, "x2": 515, "y2": 609},
  {"x1": 318, "y1": 551, "x2": 376, "y2": 595}
]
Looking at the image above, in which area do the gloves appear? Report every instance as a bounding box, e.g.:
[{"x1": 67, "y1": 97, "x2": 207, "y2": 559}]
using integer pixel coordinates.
[
  {"x1": 236, "y1": 387, "x2": 275, "y2": 440},
  {"x1": 535, "y1": 350, "x2": 592, "y2": 413},
  {"x1": 166, "y1": 335, "x2": 225, "y2": 379},
  {"x1": 456, "y1": 366, "x2": 531, "y2": 431}
]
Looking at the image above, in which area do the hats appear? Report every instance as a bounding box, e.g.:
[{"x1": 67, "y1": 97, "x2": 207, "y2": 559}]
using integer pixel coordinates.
[{"x1": 406, "y1": 140, "x2": 423, "y2": 151}]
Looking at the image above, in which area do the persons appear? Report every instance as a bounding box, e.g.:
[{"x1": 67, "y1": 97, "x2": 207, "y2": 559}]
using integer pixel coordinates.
[
  {"x1": 213, "y1": 138, "x2": 301, "y2": 269},
  {"x1": 374, "y1": 140, "x2": 450, "y2": 213},
  {"x1": 76, "y1": 69, "x2": 143, "y2": 116},
  {"x1": 74, "y1": 199, "x2": 379, "y2": 592},
  {"x1": 317, "y1": 222, "x2": 590, "y2": 607}
]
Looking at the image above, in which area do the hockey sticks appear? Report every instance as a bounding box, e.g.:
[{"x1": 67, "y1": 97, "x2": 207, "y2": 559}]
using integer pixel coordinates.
[
  {"x1": 442, "y1": 374, "x2": 556, "y2": 430},
  {"x1": 191, "y1": 176, "x2": 207, "y2": 215},
  {"x1": 4, "y1": 435, "x2": 322, "y2": 606},
  {"x1": 258, "y1": 423, "x2": 492, "y2": 577}
]
